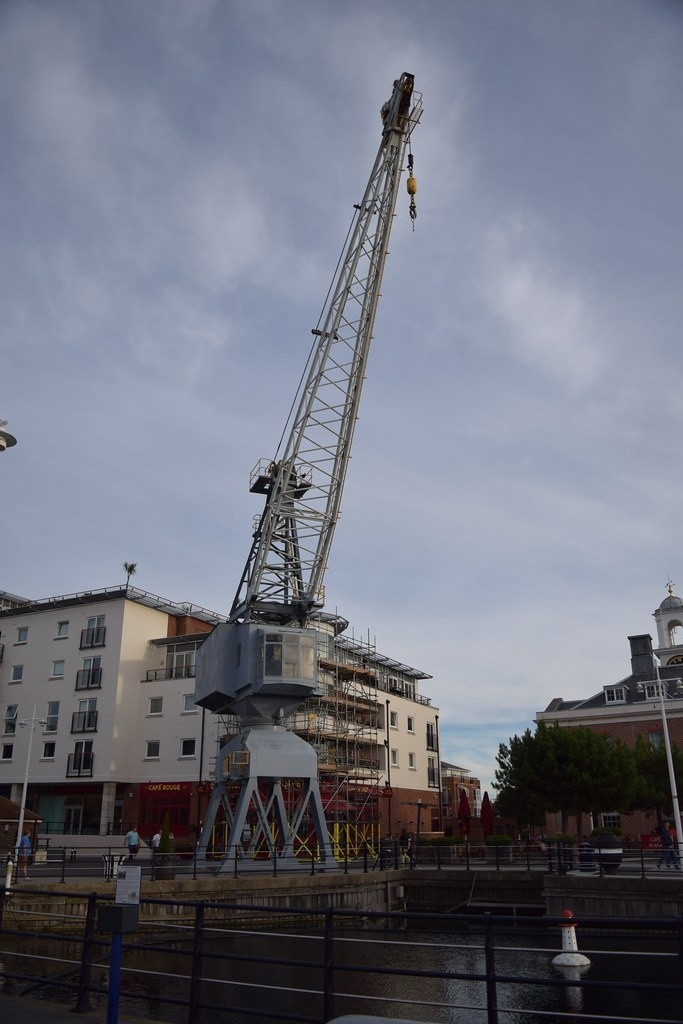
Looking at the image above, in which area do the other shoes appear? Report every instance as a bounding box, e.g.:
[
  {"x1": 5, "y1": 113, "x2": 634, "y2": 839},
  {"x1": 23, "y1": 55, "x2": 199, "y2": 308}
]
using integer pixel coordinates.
[
  {"x1": 11, "y1": 877, "x2": 15, "y2": 880},
  {"x1": 656, "y1": 864, "x2": 660, "y2": 869},
  {"x1": 24, "y1": 878, "x2": 32, "y2": 881},
  {"x1": 667, "y1": 866, "x2": 672, "y2": 869},
  {"x1": 675, "y1": 866, "x2": 681, "y2": 869}
]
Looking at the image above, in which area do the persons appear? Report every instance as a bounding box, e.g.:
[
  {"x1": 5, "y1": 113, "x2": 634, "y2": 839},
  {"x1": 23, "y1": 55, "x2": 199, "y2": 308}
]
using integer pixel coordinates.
[
  {"x1": 123, "y1": 826, "x2": 140, "y2": 859},
  {"x1": 399, "y1": 828, "x2": 414, "y2": 867},
  {"x1": 11, "y1": 830, "x2": 32, "y2": 881},
  {"x1": 656, "y1": 821, "x2": 682, "y2": 870},
  {"x1": 152, "y1": 829, "x2": 162, "y2": 853},
  {"x1": 539, "y1": 840, "x2": 546, "y2": 851}
]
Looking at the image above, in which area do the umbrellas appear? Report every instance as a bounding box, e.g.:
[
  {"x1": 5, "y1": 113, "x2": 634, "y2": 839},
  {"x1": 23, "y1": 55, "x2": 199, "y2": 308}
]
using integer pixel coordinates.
[
  {"x1": 480, "y1": 792, "x2": 495, "y2": 837},
  {"x1": 459, "y1": 790, "x2": 471, "y2": 843}
]
